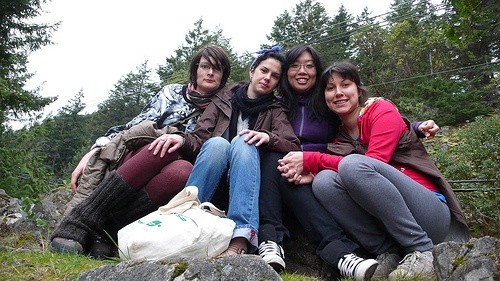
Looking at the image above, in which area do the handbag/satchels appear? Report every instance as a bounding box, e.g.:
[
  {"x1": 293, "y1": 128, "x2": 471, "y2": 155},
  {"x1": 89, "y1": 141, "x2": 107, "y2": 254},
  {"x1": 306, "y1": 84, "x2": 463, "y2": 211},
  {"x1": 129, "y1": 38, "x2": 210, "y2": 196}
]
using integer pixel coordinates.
[{"x1": 116, "y1": 185, "x2": 237, "y2": 261}]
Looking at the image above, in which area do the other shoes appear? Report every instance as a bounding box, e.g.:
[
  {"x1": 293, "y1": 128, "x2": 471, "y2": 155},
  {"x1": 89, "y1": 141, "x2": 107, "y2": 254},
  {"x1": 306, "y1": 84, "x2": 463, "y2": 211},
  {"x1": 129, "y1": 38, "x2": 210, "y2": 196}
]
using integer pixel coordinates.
[
  {"x1": 213, "y1": 245, "x2": 248, "y2": 258},
  {"x1": 48, "y1": 235, "x2": 117, "y2": 261}
]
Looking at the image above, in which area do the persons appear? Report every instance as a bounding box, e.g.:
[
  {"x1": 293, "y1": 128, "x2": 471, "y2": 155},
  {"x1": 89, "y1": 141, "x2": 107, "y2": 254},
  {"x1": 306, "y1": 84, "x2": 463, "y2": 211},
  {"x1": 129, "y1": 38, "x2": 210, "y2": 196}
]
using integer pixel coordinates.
[
  {"x1": 277, "y1": 61, "x2": 470, "y2": 281},
  {"x1": 47, "y1": 46, "x2": 231, "y2": 259},
  {"x1": 259, "y1": 46, "x2": 440, "y2": 281},
  {"x1": 147, "y1": 46, "x2": 301, "y2": 257}
]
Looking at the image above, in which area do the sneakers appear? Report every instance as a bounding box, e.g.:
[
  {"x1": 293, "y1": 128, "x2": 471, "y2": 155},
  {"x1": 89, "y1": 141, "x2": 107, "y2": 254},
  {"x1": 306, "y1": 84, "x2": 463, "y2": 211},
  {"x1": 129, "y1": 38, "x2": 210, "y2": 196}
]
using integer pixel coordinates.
[
  {"x1": 387, "y1": 250, "x2": 435, "y2": 281},
  {"x1": 337, "y1": 253, "x2": 379, "y2": 281},
  {"x1": 372, "y1": 252, "x2": 404, "y2": 279},
  {"x1": 258, "y1": 239, "x2": 286, "y2": 274}
]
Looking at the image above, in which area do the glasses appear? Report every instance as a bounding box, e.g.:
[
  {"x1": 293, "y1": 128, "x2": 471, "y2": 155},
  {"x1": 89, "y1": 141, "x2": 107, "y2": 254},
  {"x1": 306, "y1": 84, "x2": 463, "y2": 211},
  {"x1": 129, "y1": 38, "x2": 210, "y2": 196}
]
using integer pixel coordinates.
[
  {"x1": 288, "y1": 63, "x2": 316, "y2": 71},
  {"x1": 196, "y1": 63, "x2": 224, "y2": 72}
]
